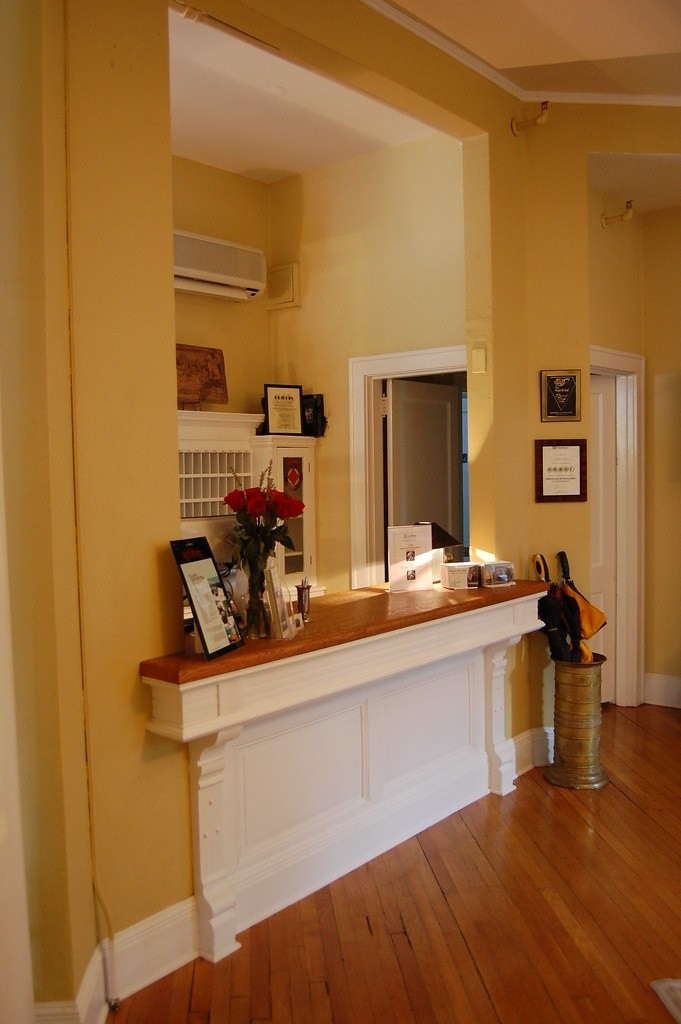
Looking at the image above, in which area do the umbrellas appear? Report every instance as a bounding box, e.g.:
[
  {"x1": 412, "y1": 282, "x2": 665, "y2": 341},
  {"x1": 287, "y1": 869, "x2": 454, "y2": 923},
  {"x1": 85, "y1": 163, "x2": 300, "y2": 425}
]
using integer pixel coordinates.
[{"x1": 530, "y1": 550, "x2": 610, "y2": 663}]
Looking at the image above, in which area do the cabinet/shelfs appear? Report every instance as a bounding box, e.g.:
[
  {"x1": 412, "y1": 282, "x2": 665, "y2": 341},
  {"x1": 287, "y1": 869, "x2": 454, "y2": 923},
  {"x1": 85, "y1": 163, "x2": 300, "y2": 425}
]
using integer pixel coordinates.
[
  {"x1": 252, "y1": 435, "x2": 324, "y2": 600},
  {"x1": 179, "y1": 449, "x2": 253, "y2": 520}
]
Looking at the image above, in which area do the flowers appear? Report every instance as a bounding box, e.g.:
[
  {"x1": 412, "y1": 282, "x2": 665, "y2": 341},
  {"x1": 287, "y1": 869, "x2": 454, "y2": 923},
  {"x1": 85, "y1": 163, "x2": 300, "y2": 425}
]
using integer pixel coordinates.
[{"x1": 221, "y1": 459, "x2": 306, "y2": 595}]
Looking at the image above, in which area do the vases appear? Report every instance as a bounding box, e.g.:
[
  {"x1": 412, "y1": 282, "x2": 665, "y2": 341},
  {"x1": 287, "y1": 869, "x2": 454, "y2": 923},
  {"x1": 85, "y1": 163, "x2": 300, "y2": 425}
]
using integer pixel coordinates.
[{"x1": 244, "y1": 595, "x2": 273, "y2": 640}]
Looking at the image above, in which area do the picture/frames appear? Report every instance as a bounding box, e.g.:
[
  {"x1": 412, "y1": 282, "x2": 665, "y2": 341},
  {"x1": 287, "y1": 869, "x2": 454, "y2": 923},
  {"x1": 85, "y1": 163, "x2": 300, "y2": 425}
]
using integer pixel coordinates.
[
  {"x1": 541, "y1": 370, "x2": 581, "y2": 422},
  {"x1": 535, "y1": 439, "x2": 587, "y2": 502},
  {"x1": 264, "y1": 384, "x2": 304, "y2": 435}
]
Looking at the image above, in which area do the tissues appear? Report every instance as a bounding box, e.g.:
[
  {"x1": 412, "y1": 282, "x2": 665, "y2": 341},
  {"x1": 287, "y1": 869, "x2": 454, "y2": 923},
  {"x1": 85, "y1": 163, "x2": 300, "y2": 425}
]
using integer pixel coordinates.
[{"x1": 476, "y1": 549, "x2": 514, "y2": 587}]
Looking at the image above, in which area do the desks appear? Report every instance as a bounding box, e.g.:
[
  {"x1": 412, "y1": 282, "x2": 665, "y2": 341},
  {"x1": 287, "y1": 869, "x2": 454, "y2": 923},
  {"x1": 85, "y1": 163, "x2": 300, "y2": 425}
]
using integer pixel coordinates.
[{"x1": 136, "y1": 568, "x2": 555, "y2": 964}]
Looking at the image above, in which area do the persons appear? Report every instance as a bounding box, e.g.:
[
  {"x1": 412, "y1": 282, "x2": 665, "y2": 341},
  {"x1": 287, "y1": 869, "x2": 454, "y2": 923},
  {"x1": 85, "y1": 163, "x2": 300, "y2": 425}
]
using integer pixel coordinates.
[{"x1": 209, "y1": 582, "x2": 240, "y2": 646}]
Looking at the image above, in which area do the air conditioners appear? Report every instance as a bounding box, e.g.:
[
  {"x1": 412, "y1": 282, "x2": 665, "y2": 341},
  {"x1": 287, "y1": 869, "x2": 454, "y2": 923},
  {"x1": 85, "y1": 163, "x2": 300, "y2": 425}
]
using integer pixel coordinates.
[{"x1": 173, "y1": 229, "x2": 267, "y2": 301}]
